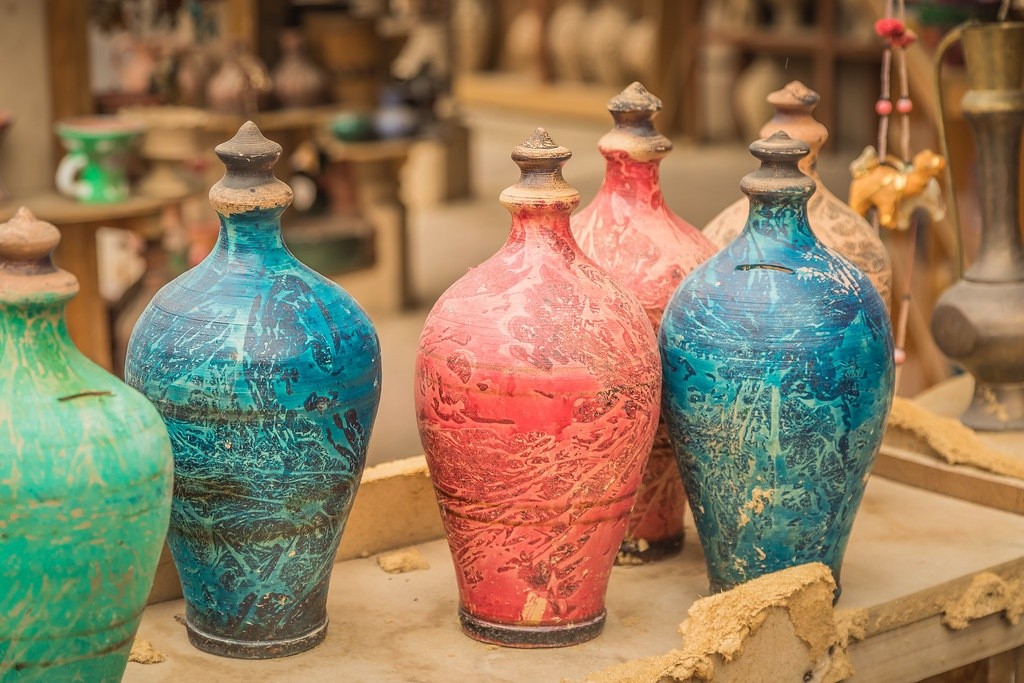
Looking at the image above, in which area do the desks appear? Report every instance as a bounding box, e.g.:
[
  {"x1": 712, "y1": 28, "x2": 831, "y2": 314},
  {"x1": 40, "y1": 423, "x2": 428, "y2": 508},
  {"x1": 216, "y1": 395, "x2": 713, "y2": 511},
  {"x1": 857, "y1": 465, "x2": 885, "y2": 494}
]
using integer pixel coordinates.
[
  {"x1": 120, "y1": 452, "x2": 1024, "y2": 683},
  {"x1": 1, "y1": 190, "x2": 186, "y2": 376},
  {"x1": 693, "y1": 24, "x2": 882, "y2": 158}
]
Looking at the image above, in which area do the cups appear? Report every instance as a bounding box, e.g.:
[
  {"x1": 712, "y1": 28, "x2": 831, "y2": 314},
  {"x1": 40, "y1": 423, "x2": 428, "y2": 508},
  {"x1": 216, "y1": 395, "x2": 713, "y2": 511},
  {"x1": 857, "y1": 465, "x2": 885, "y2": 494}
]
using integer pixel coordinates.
[{"x1": 54, "y1": 123, "x2": 144, "y2": 203}]
